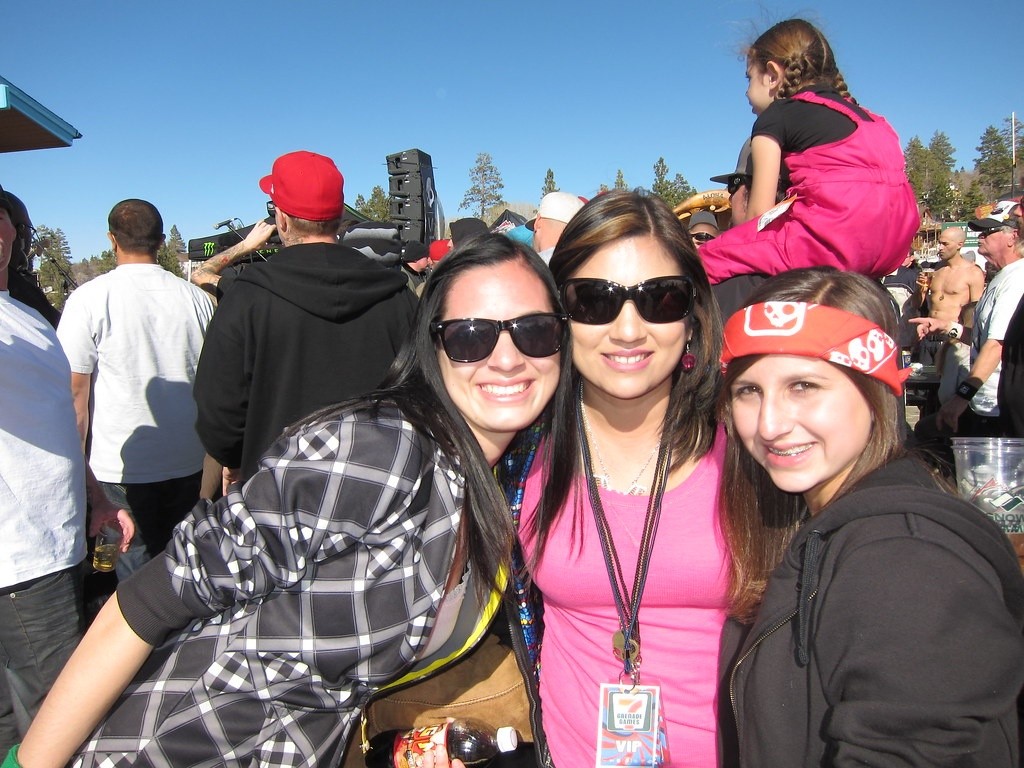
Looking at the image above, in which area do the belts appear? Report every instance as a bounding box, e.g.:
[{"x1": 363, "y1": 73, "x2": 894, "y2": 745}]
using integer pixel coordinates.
[{"x1": 927, "y1": 334, "x2": 949, "y2": 342}]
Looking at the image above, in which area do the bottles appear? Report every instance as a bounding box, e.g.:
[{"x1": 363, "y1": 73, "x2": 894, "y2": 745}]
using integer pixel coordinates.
[{"x1": 365, "y1": 716, "x2": 518, "y2": 768}]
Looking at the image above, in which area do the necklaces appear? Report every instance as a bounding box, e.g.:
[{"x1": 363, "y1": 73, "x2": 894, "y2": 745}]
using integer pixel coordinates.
[{"x1": 580, "y1": 381, "x2": 662, "y2": 496}]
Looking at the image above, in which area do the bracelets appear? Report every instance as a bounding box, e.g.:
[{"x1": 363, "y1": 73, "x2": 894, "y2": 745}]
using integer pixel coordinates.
[
  {"x1": 955, "y1": 382, "x2": 978, "y2": 401},
  {"x1": 945, "y1": 322, "x2": 962, "y2": 339}
]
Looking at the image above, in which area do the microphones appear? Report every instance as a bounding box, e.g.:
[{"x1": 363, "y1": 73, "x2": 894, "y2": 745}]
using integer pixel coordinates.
[{"x1": 214, "y1": 218, "x2": 237, "y2": 229}]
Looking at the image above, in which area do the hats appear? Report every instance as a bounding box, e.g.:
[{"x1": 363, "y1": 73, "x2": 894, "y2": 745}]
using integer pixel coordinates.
[
  {"x1": 966, "y1": 200, "x2": 1023, "y2": 233},
  {"x1": 686, "y1": 211, "x2": 718, "y2": 230},
  {"x1": 259, "y1": 149, "x2": 344, "y2": 223},
  {"x1": 524, "y1": 193, "x2": 587, "y2": 231},
  {"x1": 708, "y1": 135, "x2": 791, "y2": 183}
]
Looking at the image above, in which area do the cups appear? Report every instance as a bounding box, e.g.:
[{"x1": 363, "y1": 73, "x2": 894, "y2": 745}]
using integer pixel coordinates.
[
  {"x1": 93, "y1": 519, "x2": 129, "y2": 573},
  {"x1": 922, "y1": 268, "x2": 935, "y2": 282},
  {"x1": 950, "y1": 436, "x2": 1024, "y2": 536}
]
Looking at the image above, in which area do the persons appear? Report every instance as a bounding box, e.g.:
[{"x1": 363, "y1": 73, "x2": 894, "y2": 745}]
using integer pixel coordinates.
[
  {"x1": 717, "y1": 264, "x2": 1024, "y2": 768},
  {"x1": 697, "y1": 20, "x2": 921, "y2": 277},
  {"x1": 515, "y1": 191, "x2": 733, "y2": 768},
  {"x1": 882, "y1": 200, "x2": 1023, "y2": 474},
  {"x1": 690, "y1": 138, "x2": 791, "y2": 319},
  {"x1": 189, "y1": 220, "x2": 360, "y2": 297},
  {"x1": 193, "y1": 151, "x2": 419, "y2": 499},
  {"x1": 339, "y1": 190, "x2": 585, "y2": 298},
  {"x1": 0, "y1": 186, "x2": 135, "y2": 762},
  {"x1": 55, "y1": 198, "x2": 213, "y2": 578},
  {"x1": 0, "y1": 233, "x2": 575, "y2": 768}
]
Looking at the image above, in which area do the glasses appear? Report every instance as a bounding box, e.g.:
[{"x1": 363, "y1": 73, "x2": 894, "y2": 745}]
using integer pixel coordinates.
[
  {"x1": 727, "y1": 175, "x2": 749, "y2": 194},
  {"x1": 266, "y1": 200, "x2": 277, "y2": 219},
  {"x1": 558, "y1": 274, "x2": 696, "y2": 325},
  {"x1": 16, "y1": 223, "x2": 36, "y2": 236},
  {"x1": 983, "y1": 227, "x2": 1016, "y2": 238},
  {"x1": 691, "y1": 233, "x2": 717, "y2": 242},
  {"x1": 430, "y1": 309, "x2": 568, "y2": 362}
]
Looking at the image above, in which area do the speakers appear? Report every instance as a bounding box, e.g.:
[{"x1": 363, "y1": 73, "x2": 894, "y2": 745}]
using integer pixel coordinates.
[{"x1": 387, "y1": 148, "x2": 436, "y2": 246}]
